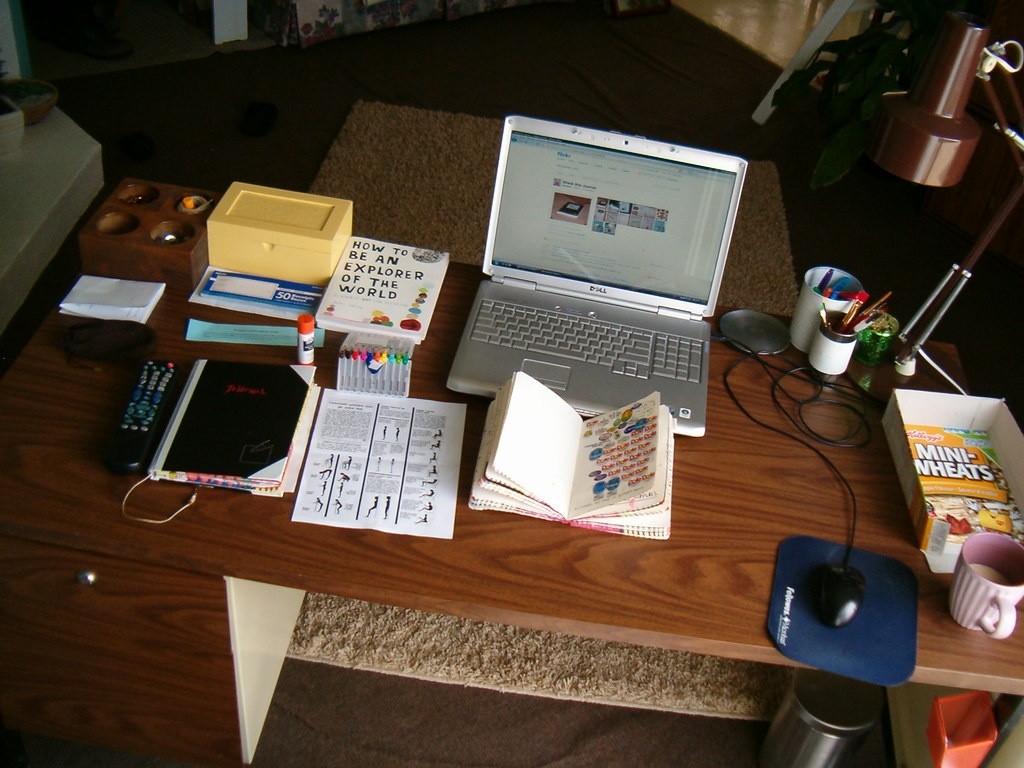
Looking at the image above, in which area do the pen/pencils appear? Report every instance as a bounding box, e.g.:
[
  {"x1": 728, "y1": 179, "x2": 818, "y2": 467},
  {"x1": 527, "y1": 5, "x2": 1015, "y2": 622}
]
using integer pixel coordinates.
[
  {"x1": 838, "y1": 292, "x2": 869, "y2": 303},
  {"x1": 823, "y1": 288, "x2": 832, "y2": 298},
  {"x1": 813, "y1": 287, "x2": 821, "y2": 294},
  {"x1": 818, "y1": 269, "x2": 834, "y2": 291},
  {"x1": 831, "y1": 277, "x2": 851, "y2": 300}
]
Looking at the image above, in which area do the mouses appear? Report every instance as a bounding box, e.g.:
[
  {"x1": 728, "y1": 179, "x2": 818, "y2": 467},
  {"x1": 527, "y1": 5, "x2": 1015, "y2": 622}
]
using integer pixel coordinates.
[{"x1": 810, "y1": 564, "x2": 866, "y2": 626}]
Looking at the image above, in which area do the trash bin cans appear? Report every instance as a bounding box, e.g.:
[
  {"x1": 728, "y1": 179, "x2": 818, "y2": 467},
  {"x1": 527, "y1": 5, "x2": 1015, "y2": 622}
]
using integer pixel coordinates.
[{"x1": 754, "y1": 666, "x2": 888, "y2": 768}]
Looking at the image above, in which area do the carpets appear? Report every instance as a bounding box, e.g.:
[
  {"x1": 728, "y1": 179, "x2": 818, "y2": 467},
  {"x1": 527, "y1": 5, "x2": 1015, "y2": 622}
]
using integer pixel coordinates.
[{"x1": 284, "y1": 97, "x2": 800, "y2": 724}]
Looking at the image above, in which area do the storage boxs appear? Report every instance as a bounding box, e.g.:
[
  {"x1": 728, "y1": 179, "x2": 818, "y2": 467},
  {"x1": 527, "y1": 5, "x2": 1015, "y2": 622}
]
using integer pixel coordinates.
[{"x1": 881, "y1": 387, "x2": 1024, "y2": 574}]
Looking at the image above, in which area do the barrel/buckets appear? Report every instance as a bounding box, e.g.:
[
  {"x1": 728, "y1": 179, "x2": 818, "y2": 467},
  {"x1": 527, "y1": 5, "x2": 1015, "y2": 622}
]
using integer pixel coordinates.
[{"x1": 758, "y1": 669, "x2": 885, "y2": 768}]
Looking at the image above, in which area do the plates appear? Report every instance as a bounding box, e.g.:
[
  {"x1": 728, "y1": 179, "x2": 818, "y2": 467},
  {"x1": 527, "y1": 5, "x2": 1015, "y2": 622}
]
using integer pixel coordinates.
[{"x1": 720, "y1": 309, "x2": 789, "y2": 354}]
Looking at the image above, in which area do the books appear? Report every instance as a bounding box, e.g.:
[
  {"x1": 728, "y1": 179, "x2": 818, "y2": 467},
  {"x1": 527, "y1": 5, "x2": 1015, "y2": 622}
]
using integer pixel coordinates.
[
  {"x1": 557, "y1": 202, "x2": 584, "y2": 218},
  {"x1": 468, "y1": 371, "x2": 676, "y2": 540},
  {"x1": 315, "y1": 236, "x2": 450, "y2": 344},
  {"x1": 147, "y1": 358, "x2": 321, "y2": 498}
]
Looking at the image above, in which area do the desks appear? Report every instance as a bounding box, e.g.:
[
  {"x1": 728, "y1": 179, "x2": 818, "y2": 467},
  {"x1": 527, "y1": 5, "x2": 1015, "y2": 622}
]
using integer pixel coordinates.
[{"x1": 0, "y1": 256, "x2": 1024, "y2": 768}]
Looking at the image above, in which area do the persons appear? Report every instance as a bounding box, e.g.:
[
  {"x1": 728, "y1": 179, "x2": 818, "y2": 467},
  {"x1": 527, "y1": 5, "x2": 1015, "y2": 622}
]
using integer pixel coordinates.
[{"x1": 20, "y1": 0, "x2": 134, "y2": 70}]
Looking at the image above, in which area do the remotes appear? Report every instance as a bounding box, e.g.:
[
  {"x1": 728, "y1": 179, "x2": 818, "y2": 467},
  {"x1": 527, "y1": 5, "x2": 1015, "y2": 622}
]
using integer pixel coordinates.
[{"x1": 110, "y1": 358, "x2": 182, "y2": 474}]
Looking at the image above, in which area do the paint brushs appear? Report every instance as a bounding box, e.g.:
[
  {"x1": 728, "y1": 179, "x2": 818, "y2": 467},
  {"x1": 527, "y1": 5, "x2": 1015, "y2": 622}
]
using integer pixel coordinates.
[
  {"x1": 844, "y1": 303, "x2": 889, "y2": 335},
  {"x1": 835, "y1": 290, "x2": 869, "y2": 333},
  {"x1": 818, "y1": 302, "x2": 827, "y2": 327},
  {"x1": 844, "y1": 291, "x2": 892, "y2": 333}
]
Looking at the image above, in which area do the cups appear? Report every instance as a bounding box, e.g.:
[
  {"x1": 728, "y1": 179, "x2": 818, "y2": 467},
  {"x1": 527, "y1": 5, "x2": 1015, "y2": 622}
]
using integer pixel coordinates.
[
  {"x1": 808, "y1": 310, "x2": 861, "y2": 375},
  {"x1": 788, "y1": 265, "x2": 865, "y2": 353},
  {"x1": 950, "y1": 532, "x2": 1024, "y2": 639}
]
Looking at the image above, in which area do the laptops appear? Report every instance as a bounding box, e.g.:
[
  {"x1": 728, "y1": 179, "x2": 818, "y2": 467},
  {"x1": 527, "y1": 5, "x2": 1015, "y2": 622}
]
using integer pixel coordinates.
[{"x1": 446, "y1": 114, "x2": 747, "y2": 435}]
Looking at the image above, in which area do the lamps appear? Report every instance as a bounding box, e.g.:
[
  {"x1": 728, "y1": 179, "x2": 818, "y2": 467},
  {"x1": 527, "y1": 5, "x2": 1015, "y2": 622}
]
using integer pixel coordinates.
[{"x1": 845, "y1": 11, "x2": 1024, "y2": 408}]
